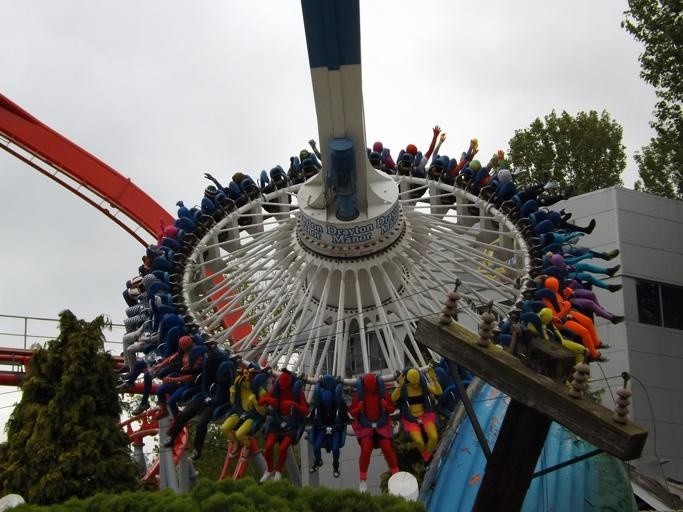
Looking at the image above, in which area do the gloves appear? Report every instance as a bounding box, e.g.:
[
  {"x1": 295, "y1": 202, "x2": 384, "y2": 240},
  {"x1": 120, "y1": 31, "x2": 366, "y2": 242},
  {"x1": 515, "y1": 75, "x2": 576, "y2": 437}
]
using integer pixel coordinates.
[
  {"x1": 427, "y1": 366, "x2": 436, "y2": 380},
  {"x1": 153, "y1": 364, "x2": 389, "y2": 412},
  {"x1": 432, "y1": 125, "x2": 504, "y2": 160},
  {"x1": 397, "y1": 374, "x2": 406, "y2": 386},
  {"x1": 563, "y1": 230, "x2": 593, "y2": 309},
  {"x1": 542, "y1": 178, "x2": 558, "y2": 188}
]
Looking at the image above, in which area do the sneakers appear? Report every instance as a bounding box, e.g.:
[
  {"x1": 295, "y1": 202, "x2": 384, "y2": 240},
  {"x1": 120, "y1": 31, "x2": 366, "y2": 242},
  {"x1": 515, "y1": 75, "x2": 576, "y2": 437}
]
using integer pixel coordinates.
[
  {"x1": 333, "y1": 467, "x2": 341, "y2": 478},
  {"x1": 132, "y1": 402, "x2": 149, "y2": 415},
  {"x1": 605, "y1": 248, "x2": 622, "y2": 293},
  {"x1": 359, "y1": 476, "x2": 368, "y2": 494},
  {"x1": 561, "y1": 184, "x2": 574, "y2": 201},
  {"x1": 113, "y1": 366, "x2": 135, "y2": 390},
  {"x1": 228, "y1": 444, "x2": 250, "y2": 461},
  {"x1": 259, "y1": 470, "x2": 282, "y2": 483},
  {"x1": 152, "y1": 403, "x2": 167, "y2": 419},
  {"x1": 594, "y1": 340, "x2": 610, "y2": 361},
  {"x1": 610, "y1": 315, "x2": 624, "y2": 325},
  {"x1": 420, "y1": 457, "x2": 430, "y2": 471},
  {"x1": 160, "y1": 438, "x2": 175, "y2": 449},
  {"x1": 187, "y1": 450, "x2": 201, "y2": 462},
  {"x1": 309, "y1": 460, "x2": 323, "y2": 473},
  {"x1": 585, "y1": 219, "x2": 597, "y2": 235}
]
